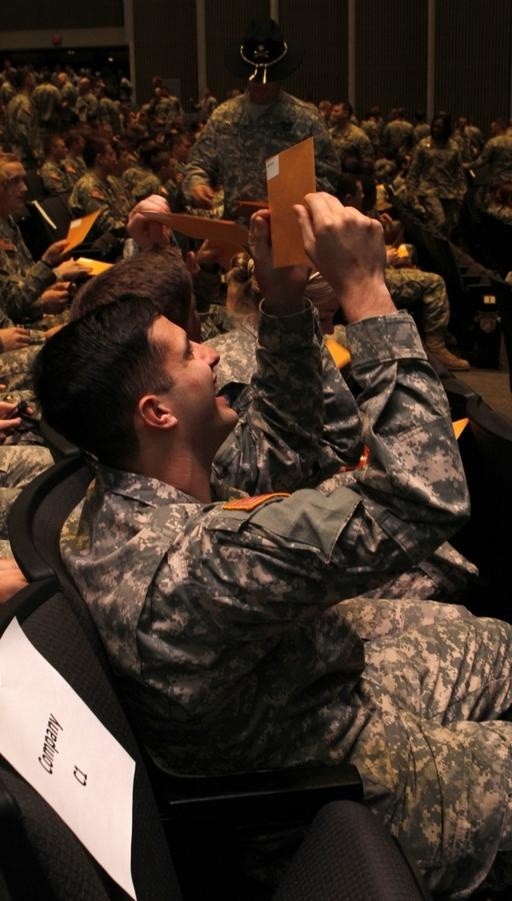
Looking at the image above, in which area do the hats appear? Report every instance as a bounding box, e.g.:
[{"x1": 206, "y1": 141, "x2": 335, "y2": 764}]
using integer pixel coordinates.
[{"x1": 225, "y1": 19, "x2": 304, "y2": 84}]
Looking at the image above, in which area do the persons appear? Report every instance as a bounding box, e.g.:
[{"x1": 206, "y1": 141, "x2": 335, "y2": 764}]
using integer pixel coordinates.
[{"x1": 0, "y1": 16, "x2": 512, "y2": 901}]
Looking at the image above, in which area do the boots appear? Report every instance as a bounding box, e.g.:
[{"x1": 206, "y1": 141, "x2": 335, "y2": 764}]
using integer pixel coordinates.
[{"x1": 428, "y1": 335, "x2": 468, "y2": 370}]
